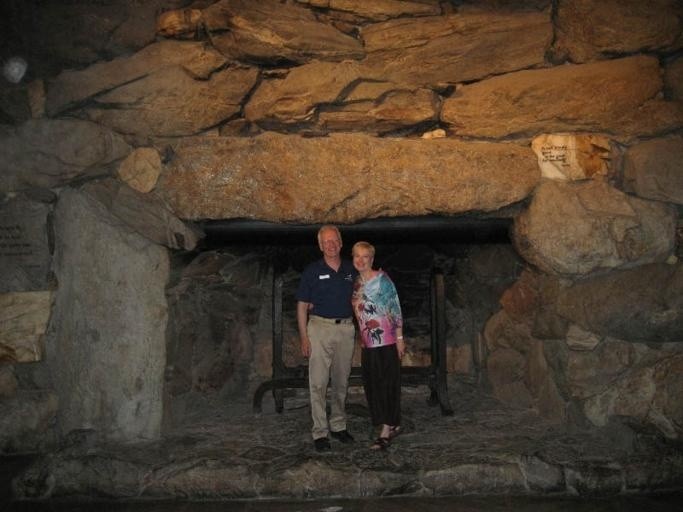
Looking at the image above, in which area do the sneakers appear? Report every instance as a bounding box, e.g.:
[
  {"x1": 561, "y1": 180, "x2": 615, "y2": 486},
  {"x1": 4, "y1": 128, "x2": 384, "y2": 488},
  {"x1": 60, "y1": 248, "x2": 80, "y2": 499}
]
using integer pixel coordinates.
[
  {"x1": 330, "y1": 430, "x2": 354, "y2": 443},
  {"x1": 314, "y1": 437, "x2": 330, "y2": 453}
]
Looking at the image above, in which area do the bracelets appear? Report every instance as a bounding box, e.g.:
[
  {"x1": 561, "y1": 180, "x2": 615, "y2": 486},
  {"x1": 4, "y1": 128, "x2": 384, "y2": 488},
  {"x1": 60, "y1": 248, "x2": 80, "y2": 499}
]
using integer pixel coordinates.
[{"x1": 396, "y1": 335, "x2": 404, "y2": 341}]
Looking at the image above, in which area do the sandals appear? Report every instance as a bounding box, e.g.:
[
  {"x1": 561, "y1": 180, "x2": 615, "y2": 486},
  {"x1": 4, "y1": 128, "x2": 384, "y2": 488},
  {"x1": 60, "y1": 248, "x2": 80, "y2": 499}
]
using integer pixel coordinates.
[{"x1": 369, "y1": 437, "x2": 392, "y2": 452}]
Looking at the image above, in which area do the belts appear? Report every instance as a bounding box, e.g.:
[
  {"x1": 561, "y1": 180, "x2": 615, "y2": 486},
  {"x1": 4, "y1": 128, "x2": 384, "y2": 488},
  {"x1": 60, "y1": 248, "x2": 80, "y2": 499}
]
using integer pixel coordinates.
[{"x1": 315, "y1": 316, "x2": 352, "y2": 325}]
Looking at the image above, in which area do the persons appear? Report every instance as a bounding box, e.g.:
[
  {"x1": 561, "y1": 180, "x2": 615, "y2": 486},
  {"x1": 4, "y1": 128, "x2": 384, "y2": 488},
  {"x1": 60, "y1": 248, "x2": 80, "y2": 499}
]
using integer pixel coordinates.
[
  {"x1": 295, "y1": 223, "x2": 361, "y2": 451},
  {"x1": 351, "y1": 241, "x2": 405, "y2": 452}
]
